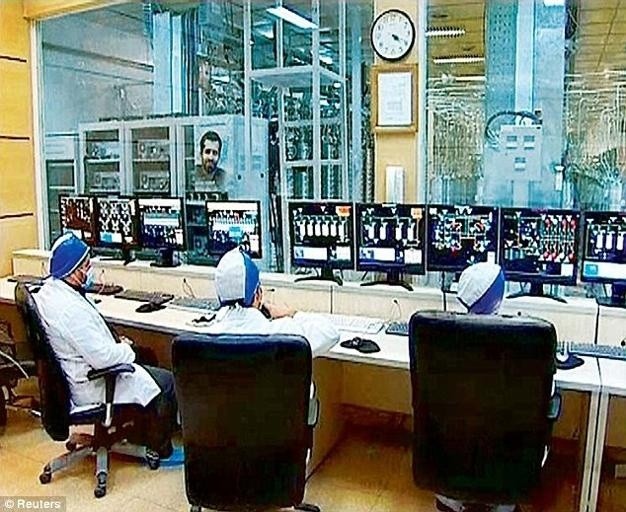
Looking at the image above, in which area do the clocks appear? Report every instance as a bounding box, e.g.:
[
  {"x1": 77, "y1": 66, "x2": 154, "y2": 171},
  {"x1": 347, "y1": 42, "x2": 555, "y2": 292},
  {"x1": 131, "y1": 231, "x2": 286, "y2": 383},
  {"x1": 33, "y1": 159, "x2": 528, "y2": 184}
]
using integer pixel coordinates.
[{"x1": 370, "y1": 9, "x2": 417, "y2": 64}]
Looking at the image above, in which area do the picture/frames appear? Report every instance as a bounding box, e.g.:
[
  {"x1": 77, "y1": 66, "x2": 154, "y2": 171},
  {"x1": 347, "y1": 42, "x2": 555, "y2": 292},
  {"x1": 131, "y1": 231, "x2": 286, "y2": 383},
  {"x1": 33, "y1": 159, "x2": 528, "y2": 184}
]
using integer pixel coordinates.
[{"x1": 370, "y1": 62, "x2": 422, "y2": 134}]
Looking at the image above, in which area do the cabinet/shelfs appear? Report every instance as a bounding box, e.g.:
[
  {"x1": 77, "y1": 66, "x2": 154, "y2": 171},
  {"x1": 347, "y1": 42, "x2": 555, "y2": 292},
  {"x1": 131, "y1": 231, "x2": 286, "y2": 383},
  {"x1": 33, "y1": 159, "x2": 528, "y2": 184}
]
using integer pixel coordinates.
[
  {"x1": 79, "y1": 114, "x2": 126, "y2": 264},
  {"x1": 175, "y1": 116, "x2": 268, "y2": 267},
  {"x1": 48, "y1": 152, "x2": 77, "y2": 250},
  {"x1": 126, "y1": 115, "x2": 175, "y2": 261}
]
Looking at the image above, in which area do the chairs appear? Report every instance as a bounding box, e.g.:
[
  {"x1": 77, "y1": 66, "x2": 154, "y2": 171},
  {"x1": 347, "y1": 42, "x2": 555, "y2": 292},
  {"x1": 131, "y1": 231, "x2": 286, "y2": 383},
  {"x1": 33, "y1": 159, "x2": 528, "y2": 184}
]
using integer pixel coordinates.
[
  {"x1": 169, "y1": 332, "x2": 324, "y2": 511},
  {"x1": 405, "y1": 308, "x2": 562, "y2": 511},
  {"x1": 13, "y1": 282, "x2": 162, "y2": 498},
  {"x1": 0, "y1": 315, "x2": 46, "y2": 433}
]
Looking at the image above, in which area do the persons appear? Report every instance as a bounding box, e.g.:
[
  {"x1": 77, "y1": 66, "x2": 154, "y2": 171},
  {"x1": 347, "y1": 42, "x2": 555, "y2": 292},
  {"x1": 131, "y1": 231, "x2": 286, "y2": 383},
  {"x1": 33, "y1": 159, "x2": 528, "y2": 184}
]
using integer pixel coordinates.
[
  {"x1": 31, "y1": 229, "x2": 185, "y2": 465},
  {"x1": 188, "y1": 130, "x2": 226, "y2": 190},
  {"x1": 434, "y1": 260, "x2": 519, "y2": 511},
  {"x1": 185, "y1": 244, "x2": 340, "y2": 399}
]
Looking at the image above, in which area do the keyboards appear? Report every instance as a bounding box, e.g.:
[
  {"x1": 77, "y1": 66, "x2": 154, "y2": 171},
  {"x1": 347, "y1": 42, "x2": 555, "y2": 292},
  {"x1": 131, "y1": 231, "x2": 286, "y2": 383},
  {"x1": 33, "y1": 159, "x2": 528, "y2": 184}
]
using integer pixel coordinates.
[{"x1": 7, "y1": 274, "x2": 626, "y2": 361}]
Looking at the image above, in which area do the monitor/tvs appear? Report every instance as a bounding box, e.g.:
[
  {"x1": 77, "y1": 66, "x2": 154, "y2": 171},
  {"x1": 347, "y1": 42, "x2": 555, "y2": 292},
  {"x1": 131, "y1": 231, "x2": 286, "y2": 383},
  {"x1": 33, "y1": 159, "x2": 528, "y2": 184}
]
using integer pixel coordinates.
[{"x1": 56, "y1": 190, "x2": 626, "y2": 309}]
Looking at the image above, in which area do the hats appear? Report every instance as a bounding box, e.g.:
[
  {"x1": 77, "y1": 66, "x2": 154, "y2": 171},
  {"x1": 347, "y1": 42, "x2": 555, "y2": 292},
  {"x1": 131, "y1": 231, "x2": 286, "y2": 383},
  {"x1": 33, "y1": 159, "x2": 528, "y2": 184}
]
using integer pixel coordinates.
[
  {"x1": 49, "y1": 232, "x2": 89, "y2": 278},
  {"x1": 215, "y1": 246, "x2": 259, "y2": 307},
  {"x1": 457, "y1": 263, "x2": 507, "y2": 314}
]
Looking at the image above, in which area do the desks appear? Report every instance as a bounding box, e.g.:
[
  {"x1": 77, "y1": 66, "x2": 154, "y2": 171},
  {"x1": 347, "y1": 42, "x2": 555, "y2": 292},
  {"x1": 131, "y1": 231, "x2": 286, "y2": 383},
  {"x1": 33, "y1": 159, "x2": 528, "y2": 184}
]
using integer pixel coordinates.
[
  {"x1": 589, "y1": 348, "x2": 626, "y2": 510},
  {"x1": 1, "y1": 248, "x2": 603, "y2": 512}
]
